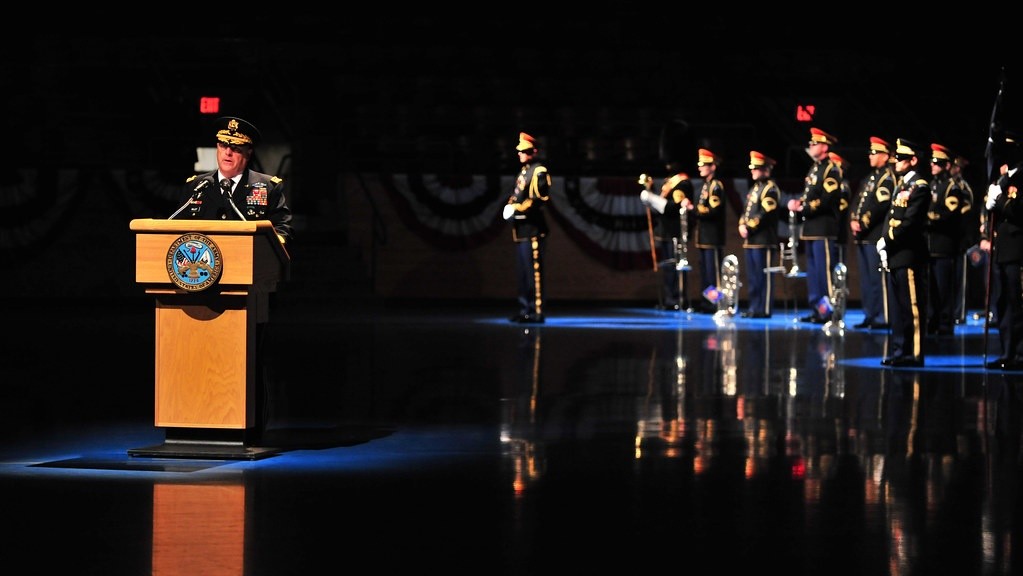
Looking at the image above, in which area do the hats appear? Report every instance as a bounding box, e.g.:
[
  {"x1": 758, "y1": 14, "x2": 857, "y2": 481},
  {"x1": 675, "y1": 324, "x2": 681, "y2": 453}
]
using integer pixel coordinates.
[
  {"x1": 869, "y1": 137, "x2": 890, "y2": 154},
  {"x1": 698, "y1": 148, "x2": 721, "y2": 167},
  {"x1": 952, "y1": 155, "x2": 968, "y2": 167},
  {"x1": 749, "y1": 150, "x2": 776, "y2": 170},
  {"x1": 515, "y1": 131, "x2": 544, "y2": 150},
  {"x1": 930, "y1": 143, "x2": 952, "y2": 161},
  {"x1": 809, "y1": 127, "x2": 837, "y2": 145},
  {"x1": 893, "y1": 138, "x2": 921, "y2": 159},
  {"x1": 214, "y1": 117, "x2": 263, "y2": 149}
]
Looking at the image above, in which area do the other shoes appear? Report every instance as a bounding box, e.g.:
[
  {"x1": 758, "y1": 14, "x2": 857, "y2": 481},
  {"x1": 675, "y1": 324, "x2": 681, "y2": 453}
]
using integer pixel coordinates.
[
  {"x1": 954, "y1": 317, "x2": 966, "y2": 324},
  {"x1": 740, "y1": 311, "x2": 771, "y2": 318},
  {"x1": 509, "y1": 312, "x2": 545, "y2": 324},
  {"x1": 984, "y1": 320, "x2": 999, "y2": 329},
  {"x1": 987, "y1": 357, "x2": 1023, "y2": 371},
  {"x1": 800, "y1": 312, "x2": 842, "y2": 324},
  {"x1": 881, "y1": 353, "x2": 924, "y2": 367},
  {"x1": 691, "y1": 297, "x2": 717, "y2": 314},
  {"x1": 853, "y1": 317, "x2": 890, "y2": 330}
]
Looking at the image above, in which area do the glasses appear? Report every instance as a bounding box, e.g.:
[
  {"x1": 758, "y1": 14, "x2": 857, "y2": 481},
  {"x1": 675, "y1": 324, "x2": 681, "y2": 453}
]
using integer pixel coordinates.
[
  {"x1": 897, "y1": 158, "x2": 904, "y2": 162},
  {"x1": 219, "y1": 142, "x2": 247, "y2": 153}
]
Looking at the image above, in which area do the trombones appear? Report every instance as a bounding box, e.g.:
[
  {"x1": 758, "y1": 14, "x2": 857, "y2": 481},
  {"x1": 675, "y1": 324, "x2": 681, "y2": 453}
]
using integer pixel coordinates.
[{"x1": 673, "y1": 207, "x2": 693, "y2": 315}]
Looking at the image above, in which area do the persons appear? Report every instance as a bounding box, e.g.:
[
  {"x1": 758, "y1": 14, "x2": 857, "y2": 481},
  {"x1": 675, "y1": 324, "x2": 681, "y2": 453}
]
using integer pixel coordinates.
[
  {"x1": 928, "y1": 143, "x2": 975, "y2": 341},
  {"x1": 851, "y1": 136, "x2": 896, "y2": 329},
  {"x1": 739, "y1": 151, "x2": 781, "y2": 318},
  {"x1": 179, "y1": 117, "x2": 294, "y2": 245},
  {"x1": 788, "y1": 128, "x2": 851, "y2": 324},
  {"x1": 980, "y1": 135, "x2": 1023, "y2": 372},
  {"x1": 503, "y1": 132, "x2": 551, "y2": 324},
  {"x1": 673, "y1": 148, "x2": 726, "y2": 313},
  {"x1": 640, "y1": 161, "x2": 696, "y2": 311},
  {"x1": 876, "y1": 138, "x2": 931, "y2": 367}
]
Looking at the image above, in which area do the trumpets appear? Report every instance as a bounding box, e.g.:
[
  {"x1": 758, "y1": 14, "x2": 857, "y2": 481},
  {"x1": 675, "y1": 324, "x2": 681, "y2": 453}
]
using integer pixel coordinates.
[
  {"x1": 780, "y1": 210, "x2": 809, "y2": 277},
  {"x1": 712, "y1": 255, "x2": 744, "y2": 322},
  {"x1": 821, "y1": 262, "x2": 849, "y2": 333}
]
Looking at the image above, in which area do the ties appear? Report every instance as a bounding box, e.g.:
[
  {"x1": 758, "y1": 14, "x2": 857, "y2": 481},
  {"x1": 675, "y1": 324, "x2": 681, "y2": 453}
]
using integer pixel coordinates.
[{"x1": 220, "y1": 178, "x2": 235, "y2": 198}]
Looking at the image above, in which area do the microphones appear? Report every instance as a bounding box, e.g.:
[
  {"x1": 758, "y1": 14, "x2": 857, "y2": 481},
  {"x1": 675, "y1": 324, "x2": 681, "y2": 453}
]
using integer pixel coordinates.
[
  {"x1": 193, "y1": 176, "x2": 215, "y2": 194},
  {"x1": 219, "y1": 178, "x2": 231, "y2": 190}
]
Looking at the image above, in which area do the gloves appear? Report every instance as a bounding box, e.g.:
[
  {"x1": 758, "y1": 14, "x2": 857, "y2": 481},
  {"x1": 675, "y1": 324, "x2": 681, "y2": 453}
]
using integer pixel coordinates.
[
  {"x1": 878, "y1": 250, "x2": 888, "y2": 267},
  {"x1": 502, "y1": 204, "x2": 514, "y2": 219},
  {"x1": 986, "y1": 199, "x2": 996, "y2": 211},
  {"x1": 876, "y1": 236, "x2": 887, "y2": 252},
  {"x1": 989, "y1": 184, "x2": 1002, "y2": 200}
]
